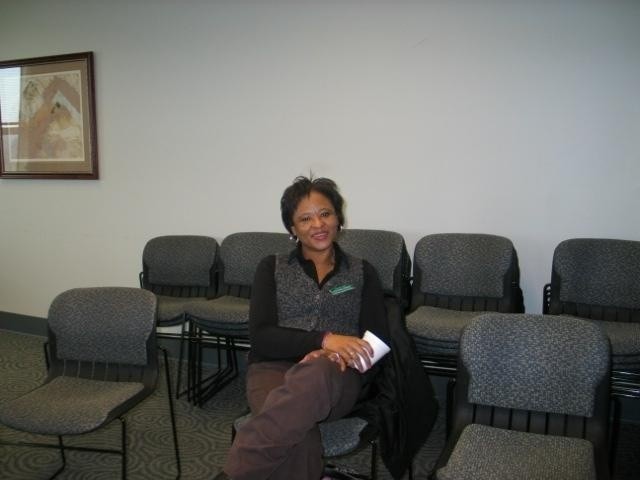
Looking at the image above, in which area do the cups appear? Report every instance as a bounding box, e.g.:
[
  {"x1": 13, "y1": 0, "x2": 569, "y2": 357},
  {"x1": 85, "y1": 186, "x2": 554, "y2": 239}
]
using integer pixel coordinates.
[{"x1": 352, "y1": 330, "x2": 391, "y2": 374}]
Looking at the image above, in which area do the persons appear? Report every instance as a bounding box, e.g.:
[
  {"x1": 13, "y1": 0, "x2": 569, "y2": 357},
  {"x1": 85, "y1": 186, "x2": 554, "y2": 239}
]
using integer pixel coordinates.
[{"x1": 212, "y1": 169, "x2": 385, "y2": 480}]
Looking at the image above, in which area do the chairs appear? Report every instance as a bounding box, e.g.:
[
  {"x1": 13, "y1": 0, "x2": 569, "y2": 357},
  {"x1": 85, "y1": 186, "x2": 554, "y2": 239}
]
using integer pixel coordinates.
[
  {"x1": 332, "y1": 228, "x2": 412, "y2": 313},
  {"x1": 229, "y1": 296, "x2": 403, "y2": 478},
  {"x1": 2, "y1": 287, "x2": 182, "y2": 479},
  {"x1": 542, "y1": 237, "x2": 639, "y2": 403},
  {"x1": 186, "y1": 231, "x2": 299, "y2": 407},
  {"x1": 134, "y1": 235, "x2": 230, "y2": 399},
  {"x1": 430, "y1": 311, "x2": 612, "y2": 480},
  {"x1": 404, "y1": 232, "x2": 523, "y2": 378}
]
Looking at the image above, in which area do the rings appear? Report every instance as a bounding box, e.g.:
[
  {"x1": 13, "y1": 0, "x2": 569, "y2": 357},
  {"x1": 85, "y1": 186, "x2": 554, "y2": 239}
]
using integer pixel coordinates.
[{"x1": 332, "y1": 352, "x2": 340, "y2": 359}]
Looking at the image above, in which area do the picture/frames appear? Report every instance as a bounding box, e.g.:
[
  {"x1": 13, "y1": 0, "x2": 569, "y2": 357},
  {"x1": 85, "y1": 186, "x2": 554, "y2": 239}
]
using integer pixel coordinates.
[{"x1": 0, "y1": 51, "x2": 100, "y2": 181}]
